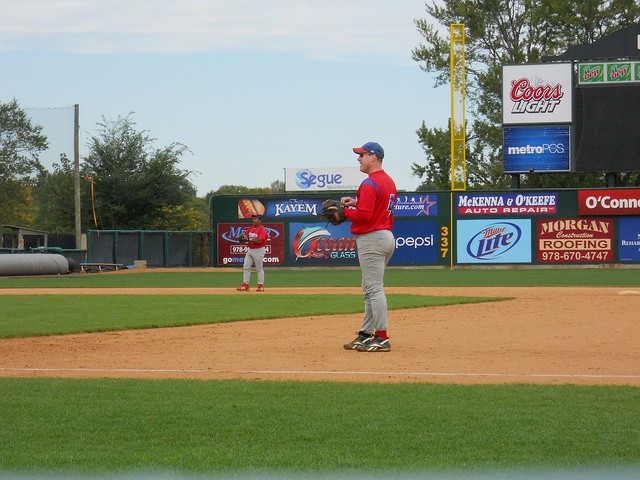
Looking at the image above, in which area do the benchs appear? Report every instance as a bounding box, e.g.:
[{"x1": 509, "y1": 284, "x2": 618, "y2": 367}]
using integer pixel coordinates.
[{"x1": 79, "y1": 263, "x2": 123, "y2": 272}]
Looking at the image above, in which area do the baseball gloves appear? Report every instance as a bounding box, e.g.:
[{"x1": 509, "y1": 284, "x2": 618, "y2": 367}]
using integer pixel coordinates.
[
  {"x1": 320, "y1": 199, "x2": 348, "y2": 226},
  {"x1": 237, "y1": 234, "x2": 249, "y2": 243}
]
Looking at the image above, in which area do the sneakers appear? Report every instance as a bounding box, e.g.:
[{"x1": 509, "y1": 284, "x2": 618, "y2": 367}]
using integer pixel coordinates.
[
  {"x1": 343, "y1": 330, "x2": 375, "y2": 350},
  {"x1": 256, "y1": 284, "x2": 264, "y2": 291},
  {"x1": 357, "y1": 335, "x2": 391, "y2": 352},
  {"x1": 237, "y1": 282, "x2": 249, "y2": 291}
]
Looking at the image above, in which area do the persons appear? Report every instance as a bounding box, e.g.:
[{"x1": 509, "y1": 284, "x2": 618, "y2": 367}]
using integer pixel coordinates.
[
  {"x1": 340, "y1": 142, "x2": 397, "y2": 353},
  {"x1": 237, "y1": 214, "x2": 268, "y2": 291}
]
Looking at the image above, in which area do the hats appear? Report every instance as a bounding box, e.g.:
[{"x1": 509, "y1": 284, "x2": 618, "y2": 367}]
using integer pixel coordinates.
[
  {"x1": 252, "y1": 214, "x2": 263, "y2": 219},
  {"x1": 353, "y1": 142, "x2": 384, "y2": 158}
]
